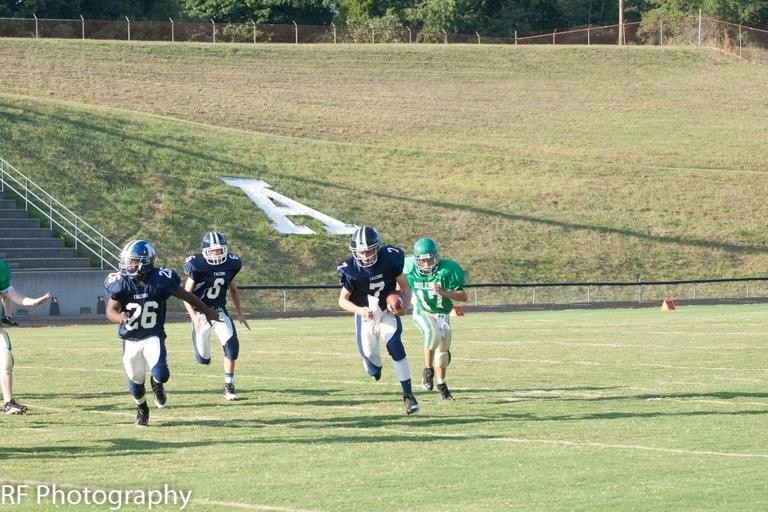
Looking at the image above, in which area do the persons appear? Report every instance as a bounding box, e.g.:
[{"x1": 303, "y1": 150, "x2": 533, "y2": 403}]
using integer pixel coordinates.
[
  {"x1": 0, "y1": 258, "x2": 52, "y2": 414},
  {"x1": 403, "y1": 238, "x2": 468, "y2": 400},
  {"x1": 337, "y1": 227, "x2": 419, "y2": 413},
  {"x1": 1, "y1": 295, "x2": 16, "y2": 325},
  {"x1": 182, "y1": 232, "x2": 251, "y2": 400},
  {"x1": 103, "y1": 239, "x2": 225, "y2": 424}
]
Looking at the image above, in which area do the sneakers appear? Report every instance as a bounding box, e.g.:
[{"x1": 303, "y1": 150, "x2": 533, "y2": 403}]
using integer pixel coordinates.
[
  {"x1": 151, "y1": 377, "x2": 167, "y2": 408},
  {"x1": 373, "y1": 367, "x2": 382, "y2": 381},
  {"x1": 422, "y1": 369, "x2": 434, "y2": 390},
  {"x1": 136, "y1": 405, "x2": 149, "y2": 426},
  {"x1": 404, "y1": 395, "x2": 418, "y2": 416},
  {"x1": 437, "y1": 383, "x2": 452, "y2": 401},
  {"x1": 4, "y1": 399, "x2": 26, "y2": 414},
  {"x1": 224, "y1": 383, "x2": 237, "y2": 400}
]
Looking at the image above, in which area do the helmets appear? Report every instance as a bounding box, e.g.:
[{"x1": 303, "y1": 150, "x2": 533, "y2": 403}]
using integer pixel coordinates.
[
  {"x1": 412, "y1": 238, "x2": 439, "y2": 276},
  {"x1": 119, "y1": 240, "x2": 155, "y2": 277},
  {"x1": 349, "y1": 227, "x2": 380, "y2": 268},
  {"x1": 202, "y1": 232, "x2": 228, "y2": 265}
]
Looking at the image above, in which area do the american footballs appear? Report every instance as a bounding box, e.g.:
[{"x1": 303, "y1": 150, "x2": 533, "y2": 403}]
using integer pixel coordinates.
[{"x1": 386, "y1": 289, "x2": 405, "y2": 313}]
